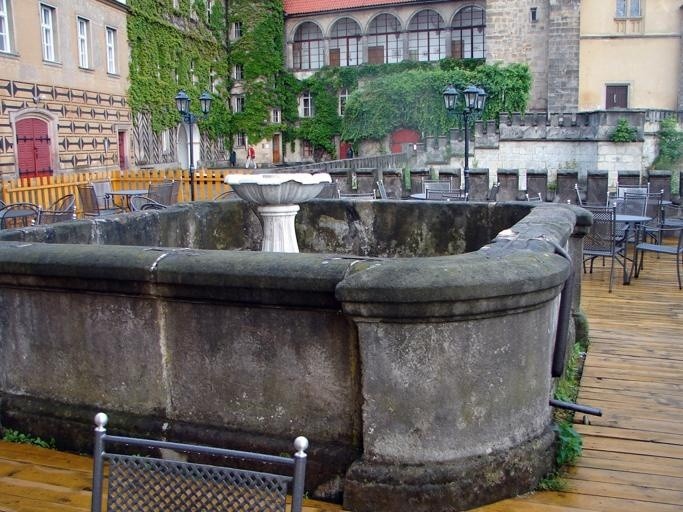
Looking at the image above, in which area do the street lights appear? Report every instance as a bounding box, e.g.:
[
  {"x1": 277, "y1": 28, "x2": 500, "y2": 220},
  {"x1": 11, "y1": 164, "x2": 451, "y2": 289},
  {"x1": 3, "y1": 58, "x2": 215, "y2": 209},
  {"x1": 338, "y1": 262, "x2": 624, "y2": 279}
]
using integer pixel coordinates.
[
  {"x1": 173, "y1": 88, "x2": 215, "y2": 203},
  {"x1": 443, "y1": 82, "x2": 488, "y2": 173}
]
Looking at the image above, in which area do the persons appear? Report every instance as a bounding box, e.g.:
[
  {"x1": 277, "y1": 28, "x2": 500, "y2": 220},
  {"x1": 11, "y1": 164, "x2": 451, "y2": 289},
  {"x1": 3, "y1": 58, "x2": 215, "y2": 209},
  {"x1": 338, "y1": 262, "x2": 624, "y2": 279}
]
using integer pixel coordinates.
[
  {"x1": 347, "y1": 146, "x2": 353, "y2": 158},
  {"x1": 246, "y1": 145, "x2": 256, "y2": 169}
]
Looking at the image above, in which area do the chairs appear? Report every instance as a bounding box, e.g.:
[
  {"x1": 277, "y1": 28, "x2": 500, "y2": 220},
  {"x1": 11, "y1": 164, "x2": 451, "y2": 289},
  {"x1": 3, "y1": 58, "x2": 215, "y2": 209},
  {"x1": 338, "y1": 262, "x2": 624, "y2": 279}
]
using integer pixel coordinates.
[
  {"x1": 0, "y1": 178, "x2": 375, "y2": 229},
  {"x1": 524, "y1": 179, "x2": 682, "y2": 292},
  {"x1": 92, "y1": 412, "x2": 309, "y2": 512},
  {"x1": 378, "y1": 180, "x2": 525, "y2": 204}
]
